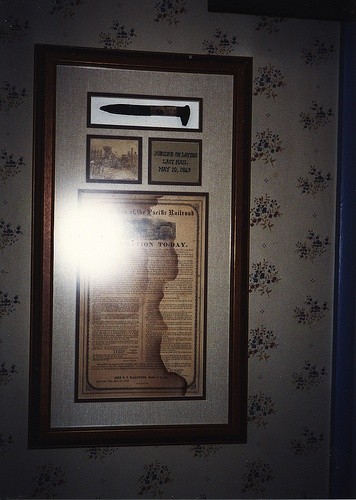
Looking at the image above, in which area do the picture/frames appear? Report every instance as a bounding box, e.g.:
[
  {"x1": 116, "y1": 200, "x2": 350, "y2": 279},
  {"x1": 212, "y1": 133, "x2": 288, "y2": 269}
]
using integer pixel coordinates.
[{"x1": 24, "y1": 41, "x2": 254, "y2": 449}]
